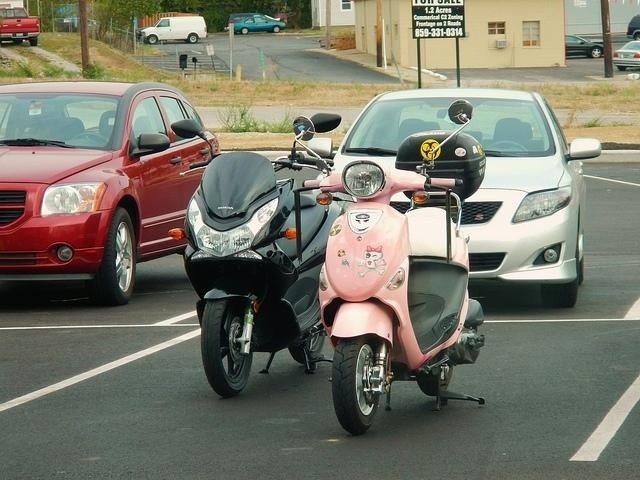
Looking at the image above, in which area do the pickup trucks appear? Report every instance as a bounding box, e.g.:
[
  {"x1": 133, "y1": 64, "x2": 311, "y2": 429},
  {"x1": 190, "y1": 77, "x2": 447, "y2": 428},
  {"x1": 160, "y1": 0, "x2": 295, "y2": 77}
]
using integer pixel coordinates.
[{"x1": 0, "y1": 7, "x2": 40, "y2": 47}]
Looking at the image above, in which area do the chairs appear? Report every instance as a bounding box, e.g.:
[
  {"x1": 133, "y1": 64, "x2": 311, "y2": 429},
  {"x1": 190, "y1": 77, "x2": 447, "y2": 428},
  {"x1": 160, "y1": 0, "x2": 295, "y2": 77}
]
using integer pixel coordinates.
[
  {"x1": 96, "y1": 109, "x2": 134, "y2": 142},
  {"x1": 520, "y1": 122, "x2": 533, "y2": 141},
  {"x1": 133, "y1": 118, "x2": 154, "y2": 138},
  {"x1": 50, "y1": 117, "x2": 84, "y2": 140},
  {"x1": 397, "y1": 116, "x2": 426, "y2": 146},
  {"x1": 491, "y1": 118, "x2": 526, "y2": 150},
  {"x1": 424, "y1": 121, "x2": 442, "y2": 132}
]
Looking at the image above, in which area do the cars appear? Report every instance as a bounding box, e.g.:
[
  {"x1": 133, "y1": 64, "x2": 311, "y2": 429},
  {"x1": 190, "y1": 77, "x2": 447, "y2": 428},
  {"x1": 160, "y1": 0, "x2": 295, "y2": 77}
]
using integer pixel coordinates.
[
  {"x1": 626, "y1": 14, "x2": 640, "y2": 40},
  {"x1": 305, "y1": 86, "x2": 603, "y2": 310},
  {"x1": 612, "y1": 40, "x2": 640, "y2": 71},
  {"x1": 565, "y1": 33, "x2": 603, "y2": 59},
  {"x1": 1, "y1": 78, "x2": 223, "y2": 307},
  {"x1": 39, "y1": 3, "x2": 97, "y2": 33}
]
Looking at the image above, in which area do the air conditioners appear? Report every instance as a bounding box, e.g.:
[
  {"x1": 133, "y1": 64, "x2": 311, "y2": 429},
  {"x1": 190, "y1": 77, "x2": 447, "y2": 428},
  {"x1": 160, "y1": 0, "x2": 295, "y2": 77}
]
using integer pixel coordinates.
[{"x1": 496, "y1": 39, "x2": 508, "y2": 50}]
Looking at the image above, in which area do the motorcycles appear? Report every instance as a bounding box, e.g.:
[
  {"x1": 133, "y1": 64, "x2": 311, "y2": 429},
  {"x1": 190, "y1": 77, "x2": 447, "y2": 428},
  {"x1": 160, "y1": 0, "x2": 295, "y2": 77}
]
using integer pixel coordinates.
[
  {"x1": 166, "y1": 111, "x2": 343, "y2": 404},
  {"x1": 290, "y1": 100, "x2": 486, "y2": 435}
]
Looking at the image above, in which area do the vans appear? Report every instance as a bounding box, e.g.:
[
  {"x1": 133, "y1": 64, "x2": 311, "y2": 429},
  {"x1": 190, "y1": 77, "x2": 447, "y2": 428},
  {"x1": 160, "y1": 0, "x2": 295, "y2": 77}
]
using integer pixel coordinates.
[
  {"x1": 224, "y1": 14, "x2": 288, "y2": 35},
  {"x1": 138, "y1": 16, "x2": 209, "y2": 46}
]
